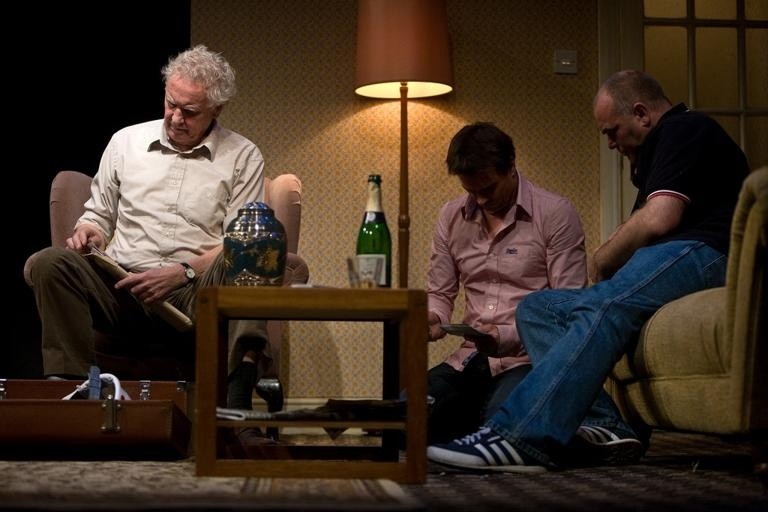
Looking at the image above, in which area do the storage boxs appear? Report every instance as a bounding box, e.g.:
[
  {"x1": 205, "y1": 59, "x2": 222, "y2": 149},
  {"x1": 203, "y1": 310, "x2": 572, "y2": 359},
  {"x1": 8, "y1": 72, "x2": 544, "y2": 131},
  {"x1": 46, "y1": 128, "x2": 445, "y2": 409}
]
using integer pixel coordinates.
[{"x1": 1, "y1": 378, "x2": 191, "y2": 460}]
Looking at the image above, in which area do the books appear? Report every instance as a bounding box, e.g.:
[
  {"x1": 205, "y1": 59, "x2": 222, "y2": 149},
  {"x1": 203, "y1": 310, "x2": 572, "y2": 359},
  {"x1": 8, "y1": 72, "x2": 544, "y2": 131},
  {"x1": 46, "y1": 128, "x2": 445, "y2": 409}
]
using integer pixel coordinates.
[{"x1": 84, "y1": 242, "x2": 193, "y2": 332}]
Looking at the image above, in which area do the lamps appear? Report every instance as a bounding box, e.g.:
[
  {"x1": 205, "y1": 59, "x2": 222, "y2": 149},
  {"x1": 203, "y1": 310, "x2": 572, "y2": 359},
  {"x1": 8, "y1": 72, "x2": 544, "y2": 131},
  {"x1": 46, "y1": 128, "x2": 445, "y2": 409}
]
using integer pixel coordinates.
[{"x1": 353, "y1": 0, "x2": 454, "y2": 290}]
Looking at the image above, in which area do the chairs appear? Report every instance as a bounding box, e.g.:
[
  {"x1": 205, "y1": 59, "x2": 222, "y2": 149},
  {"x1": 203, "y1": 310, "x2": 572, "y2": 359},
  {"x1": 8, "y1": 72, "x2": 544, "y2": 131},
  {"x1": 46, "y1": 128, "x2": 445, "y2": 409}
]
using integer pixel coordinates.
[
  {"x1": 600, "y1": 166, "x2": 768, "y2": 482},
  {"x1": 23, "y1": 170, "x2": 309, "y2": 441}
]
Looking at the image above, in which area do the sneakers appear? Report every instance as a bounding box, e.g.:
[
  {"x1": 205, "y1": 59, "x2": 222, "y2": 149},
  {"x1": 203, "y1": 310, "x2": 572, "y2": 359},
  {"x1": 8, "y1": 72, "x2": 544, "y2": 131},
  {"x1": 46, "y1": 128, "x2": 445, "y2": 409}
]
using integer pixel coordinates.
[
  {"x1": 545, "y1": 422, "x2": 645, "y2": 465},
  {"x1": 427, "y1": 424, "x2": 550, "y2": 477}
]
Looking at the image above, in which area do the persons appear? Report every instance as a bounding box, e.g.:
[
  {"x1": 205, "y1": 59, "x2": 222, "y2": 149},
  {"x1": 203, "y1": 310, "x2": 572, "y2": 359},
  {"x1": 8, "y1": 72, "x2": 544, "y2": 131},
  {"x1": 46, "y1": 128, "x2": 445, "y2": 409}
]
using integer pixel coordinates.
[
  {"x1": 22, "y1": 44, "x2": 270, "y2": 404},
  {"x1": 429, "y1": 68, "x2": 751, "y2": 474},
  {"x1": 427, "y1": 121, "x2": 589, "y2": 438}
]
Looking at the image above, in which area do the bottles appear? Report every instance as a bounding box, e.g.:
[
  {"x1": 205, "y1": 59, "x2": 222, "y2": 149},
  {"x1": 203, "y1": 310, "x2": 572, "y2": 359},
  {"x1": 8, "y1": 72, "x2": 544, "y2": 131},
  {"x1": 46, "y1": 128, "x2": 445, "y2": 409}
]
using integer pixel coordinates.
[{"x1": 355, "y1": 173, "x2": 392, "y2": 290}]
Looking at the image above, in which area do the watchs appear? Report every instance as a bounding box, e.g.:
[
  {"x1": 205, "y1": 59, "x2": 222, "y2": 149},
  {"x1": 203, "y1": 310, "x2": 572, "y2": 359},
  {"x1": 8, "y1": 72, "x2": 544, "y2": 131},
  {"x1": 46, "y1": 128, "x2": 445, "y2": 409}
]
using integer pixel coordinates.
[{"x1": 180, "y1": 262, "x2": 196, "y2": 282}]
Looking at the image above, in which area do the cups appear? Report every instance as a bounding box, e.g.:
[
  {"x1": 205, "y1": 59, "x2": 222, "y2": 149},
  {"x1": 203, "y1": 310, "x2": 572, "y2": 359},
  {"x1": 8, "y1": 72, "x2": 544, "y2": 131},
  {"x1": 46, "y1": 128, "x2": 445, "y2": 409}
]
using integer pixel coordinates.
[{"x1": 347, "y1": 255, "x2": 384, "y2": 291}]
